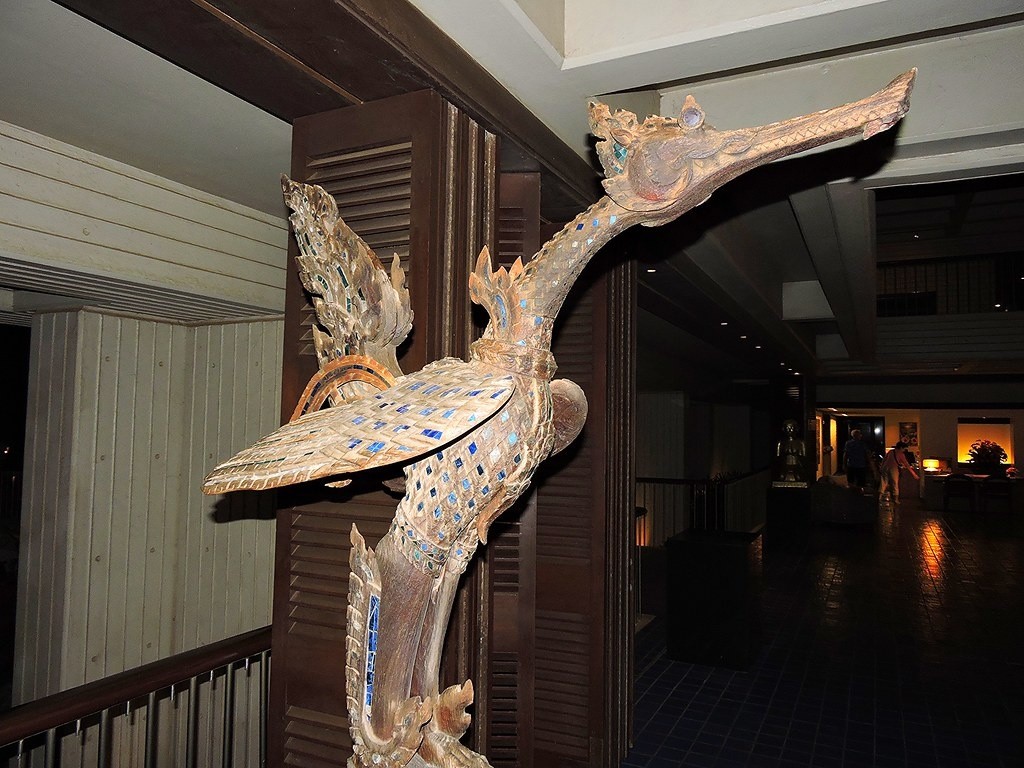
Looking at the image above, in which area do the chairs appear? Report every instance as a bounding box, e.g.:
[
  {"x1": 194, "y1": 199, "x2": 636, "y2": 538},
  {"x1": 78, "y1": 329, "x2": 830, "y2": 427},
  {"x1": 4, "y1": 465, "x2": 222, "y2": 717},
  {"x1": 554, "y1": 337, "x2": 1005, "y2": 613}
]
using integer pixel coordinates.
[{"x1": 942, "y1": 473, "x2": 1012, "y2": 525}]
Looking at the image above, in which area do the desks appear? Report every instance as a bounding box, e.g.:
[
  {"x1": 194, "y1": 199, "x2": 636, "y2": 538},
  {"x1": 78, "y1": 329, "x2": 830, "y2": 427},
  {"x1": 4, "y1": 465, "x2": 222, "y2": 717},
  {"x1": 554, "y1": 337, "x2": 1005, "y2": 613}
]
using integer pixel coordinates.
[{"x1": 926, "y1": 473, "x2": 1024, "y2": 510}]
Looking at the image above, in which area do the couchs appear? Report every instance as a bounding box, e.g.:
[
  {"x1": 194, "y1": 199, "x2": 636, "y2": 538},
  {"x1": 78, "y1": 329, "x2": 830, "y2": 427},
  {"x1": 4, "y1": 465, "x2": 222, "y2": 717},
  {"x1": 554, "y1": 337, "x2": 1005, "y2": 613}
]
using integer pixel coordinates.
[{"x1": 812, "y1": 474, "x2": 876, "y2": 524}]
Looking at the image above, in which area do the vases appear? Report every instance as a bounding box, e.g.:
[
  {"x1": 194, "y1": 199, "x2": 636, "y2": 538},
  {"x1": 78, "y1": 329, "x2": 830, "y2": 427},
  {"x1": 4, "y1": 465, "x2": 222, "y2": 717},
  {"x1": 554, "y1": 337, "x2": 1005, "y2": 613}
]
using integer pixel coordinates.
[{"x1": 976, "y1": 458, "x2": 1000, "y2": 466}]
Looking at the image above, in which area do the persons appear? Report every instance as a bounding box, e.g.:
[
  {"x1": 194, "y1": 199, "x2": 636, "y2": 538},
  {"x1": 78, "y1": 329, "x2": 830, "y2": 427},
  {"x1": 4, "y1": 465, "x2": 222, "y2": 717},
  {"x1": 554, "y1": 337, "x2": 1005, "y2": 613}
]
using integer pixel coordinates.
[
  {"x1": 843, "y1": 429, "x2": 871, "y2": 495},
  {"x1": 878, "y1": 441, "x2": 919, "y2": 504},
  {"x1": 777, "y1": 420, "x2": 808, "y2": 481}
]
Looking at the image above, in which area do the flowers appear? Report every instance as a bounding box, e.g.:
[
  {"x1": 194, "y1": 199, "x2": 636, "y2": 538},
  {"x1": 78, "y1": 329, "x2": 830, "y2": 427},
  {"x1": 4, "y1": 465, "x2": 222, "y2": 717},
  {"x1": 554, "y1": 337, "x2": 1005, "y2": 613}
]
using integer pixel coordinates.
[{"x1": 969, "y1": 439, "x2": 1007, "y2": 459}]
